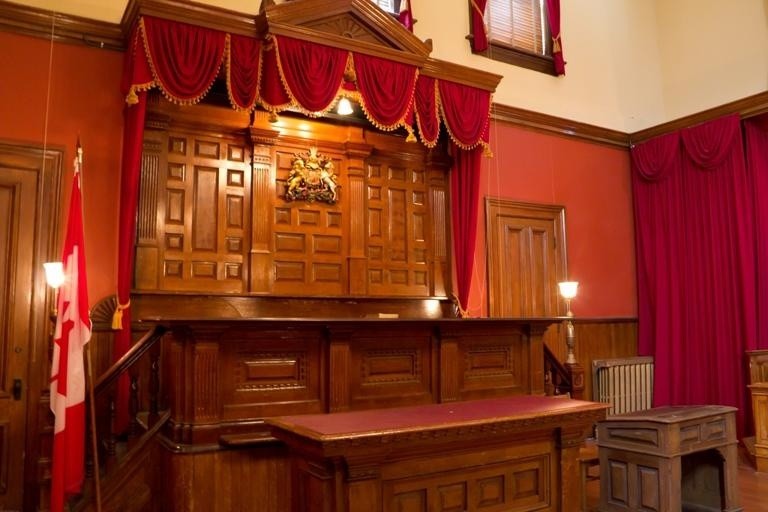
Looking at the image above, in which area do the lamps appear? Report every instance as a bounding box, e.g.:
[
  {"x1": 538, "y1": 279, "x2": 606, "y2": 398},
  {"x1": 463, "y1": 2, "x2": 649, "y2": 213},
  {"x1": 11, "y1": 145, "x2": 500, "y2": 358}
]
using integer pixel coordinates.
[
  {"x1": 43, "y1": 260, "x2": 64, "y2": 323},
  {"x1": 559, "y1": 281, "x2": 579, "y2": 364}
]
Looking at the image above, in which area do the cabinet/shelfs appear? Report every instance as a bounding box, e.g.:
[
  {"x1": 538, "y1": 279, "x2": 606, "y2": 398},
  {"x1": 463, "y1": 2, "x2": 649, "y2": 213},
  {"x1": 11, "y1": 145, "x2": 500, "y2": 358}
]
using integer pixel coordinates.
[
  {"x1": 596, "y1": 402, "x2": 740, "y2": 512},
  {"x1": 264, "y1": 393, "x2": 613, "y2": 509}
]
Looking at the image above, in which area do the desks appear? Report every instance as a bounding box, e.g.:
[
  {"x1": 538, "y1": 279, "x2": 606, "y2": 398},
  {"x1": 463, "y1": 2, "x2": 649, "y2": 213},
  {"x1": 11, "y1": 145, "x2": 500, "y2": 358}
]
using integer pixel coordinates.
[{"x1": 746, "y1": 347, "x2": 768, "y2": 472}]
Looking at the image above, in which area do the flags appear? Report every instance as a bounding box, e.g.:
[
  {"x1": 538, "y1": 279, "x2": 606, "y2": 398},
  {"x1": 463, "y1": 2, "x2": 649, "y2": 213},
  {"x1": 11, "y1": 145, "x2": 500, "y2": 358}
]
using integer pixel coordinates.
[{"x1": 49, "y1": 157, "x2": 92, "y2": 512}]
